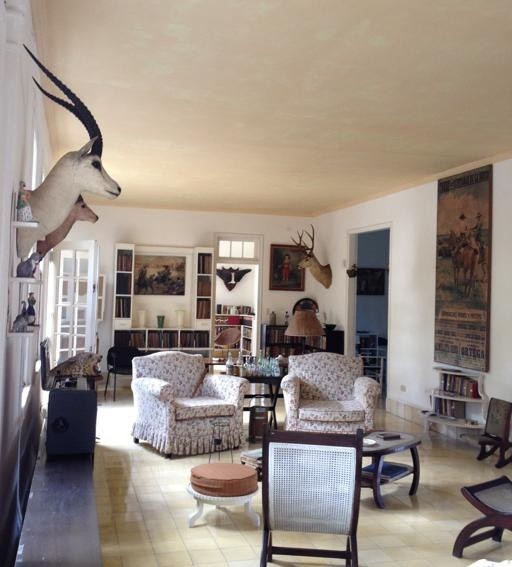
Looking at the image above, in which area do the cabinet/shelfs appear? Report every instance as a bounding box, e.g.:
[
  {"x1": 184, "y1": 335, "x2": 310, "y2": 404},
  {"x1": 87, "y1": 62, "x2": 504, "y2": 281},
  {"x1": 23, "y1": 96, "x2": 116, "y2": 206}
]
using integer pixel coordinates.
[
  {"x1": 6, "y1": 219, "x2": 43, "y2": 338},
  {"x1": 212, "y1": 314, "x2": 254, "y2": 357},
  {"x1": 417, "y1": 365, "x2": 487, "y2": 440},
  {"x1": 110, "y1": 242, "x2": 213, "y2": 351},
  {"x1": 261, "y1": 323, "x2": 344, "y2": 357}
]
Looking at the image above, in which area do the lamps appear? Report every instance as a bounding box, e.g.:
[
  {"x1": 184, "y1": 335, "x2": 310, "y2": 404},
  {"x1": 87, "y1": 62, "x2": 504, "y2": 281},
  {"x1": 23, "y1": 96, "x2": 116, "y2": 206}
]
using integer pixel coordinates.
[{"x1": 283, "y1": 309, "x2": 326, "y2": 355}]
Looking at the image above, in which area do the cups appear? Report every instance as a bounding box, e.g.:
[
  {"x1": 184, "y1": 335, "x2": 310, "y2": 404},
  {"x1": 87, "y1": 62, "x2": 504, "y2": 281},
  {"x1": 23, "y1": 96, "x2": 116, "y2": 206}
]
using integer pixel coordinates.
[
  {"x1": 229, "y1": 308, "x2": 239, "y2": 314},
  {"x1": 157, "y1": 316, "x2": 164, "y2": 327},
  {"x1": 138, "y1": 310, "x2": 145, "y2": 328},
  {"x1": 177, "y1": 310, "x2": 185, "y2": 329}
]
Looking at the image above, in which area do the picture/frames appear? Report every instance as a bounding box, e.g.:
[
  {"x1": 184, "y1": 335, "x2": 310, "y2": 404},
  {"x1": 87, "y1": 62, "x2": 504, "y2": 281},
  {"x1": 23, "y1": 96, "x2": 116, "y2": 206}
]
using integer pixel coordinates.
[
  {"x1": 357, "y1": 266, "x2": 386, "y2": 295},
  {"x1": 268, "y1": 243, "x2": 306, "y2": 292}
]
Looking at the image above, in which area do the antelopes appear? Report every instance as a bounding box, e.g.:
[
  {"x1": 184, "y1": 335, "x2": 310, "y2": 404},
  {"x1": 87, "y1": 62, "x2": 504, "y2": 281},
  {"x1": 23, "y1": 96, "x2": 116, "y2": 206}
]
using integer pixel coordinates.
[
  {"x1": 13, "y1": 39, "x2": 123, "y2": 256},
  {"x1": 31, "y1": 195, "x2": 100, "y2": 261}
]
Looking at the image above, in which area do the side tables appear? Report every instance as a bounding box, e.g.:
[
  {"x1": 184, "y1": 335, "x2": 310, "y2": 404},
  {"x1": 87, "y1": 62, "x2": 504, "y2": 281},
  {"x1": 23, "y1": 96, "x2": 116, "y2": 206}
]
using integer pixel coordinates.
[{"x1": 220, "y1": 371, "x2": 286, "y2": 430}]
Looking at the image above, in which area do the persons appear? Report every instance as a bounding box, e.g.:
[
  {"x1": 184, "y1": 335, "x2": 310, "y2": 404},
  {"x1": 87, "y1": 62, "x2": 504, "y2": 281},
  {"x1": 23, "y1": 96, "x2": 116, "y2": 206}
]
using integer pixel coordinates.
[{"x1": 278, "y1": 254, "x2": 292, "y2": 281}]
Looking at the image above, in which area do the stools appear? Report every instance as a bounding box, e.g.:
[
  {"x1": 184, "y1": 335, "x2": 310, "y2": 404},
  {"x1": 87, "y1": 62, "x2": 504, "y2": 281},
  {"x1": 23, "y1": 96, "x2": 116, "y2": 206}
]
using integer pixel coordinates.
[{"x1": 186, "y1": 463, "x2": 262, "y2": 530}]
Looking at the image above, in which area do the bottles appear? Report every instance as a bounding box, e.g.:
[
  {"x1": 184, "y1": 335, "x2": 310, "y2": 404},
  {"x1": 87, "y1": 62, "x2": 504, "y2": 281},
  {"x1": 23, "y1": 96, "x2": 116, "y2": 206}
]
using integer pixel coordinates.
[
  {"x1": 268, "y1": 311, "x2": 277, "y2": 326},
  {"x1": 25, "y1": 293, "x2": 35, "y2": 325},
  {"x1": 284, "y1": 311, "x2": 289, "y2": 326},
  {"x1": 224, "y1": 350, "x2": 280, "y2": 377}
]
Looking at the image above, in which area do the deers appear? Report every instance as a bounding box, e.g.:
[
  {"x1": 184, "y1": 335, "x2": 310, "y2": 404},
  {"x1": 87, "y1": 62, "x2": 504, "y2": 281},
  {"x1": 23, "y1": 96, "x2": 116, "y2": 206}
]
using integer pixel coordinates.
[{"x1": 290, "y1": 223, "x2": 332, "y2": 289}]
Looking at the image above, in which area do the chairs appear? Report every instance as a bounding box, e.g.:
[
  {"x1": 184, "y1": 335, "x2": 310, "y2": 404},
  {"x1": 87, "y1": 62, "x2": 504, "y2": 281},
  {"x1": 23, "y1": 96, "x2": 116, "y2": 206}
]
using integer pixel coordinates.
[
  {"x1": 103, "y1": 344, "x2": 140, "y2": 401},
  {"x1": 464, "y1": 396, "x2": 512, "y2": 468},
  {"x1": 215, "y1": 327, "x2": 242, "y2": 356},
  {"x1": 280, "y1": 351, "x2": 382, "y2": 435},
  {"x1": 259, "y1": 422, "x2": 365, "y2": 564},
  {"x1": 129, "y1": 351, "x2": 250, "y2": 459}
]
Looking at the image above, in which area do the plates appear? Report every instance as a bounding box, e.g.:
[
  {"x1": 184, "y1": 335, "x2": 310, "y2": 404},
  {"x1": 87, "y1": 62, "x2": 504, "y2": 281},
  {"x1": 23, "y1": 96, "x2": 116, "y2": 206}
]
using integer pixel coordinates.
[{"x1": 362, "y1": 438, "x2": 377, "y2": 446}]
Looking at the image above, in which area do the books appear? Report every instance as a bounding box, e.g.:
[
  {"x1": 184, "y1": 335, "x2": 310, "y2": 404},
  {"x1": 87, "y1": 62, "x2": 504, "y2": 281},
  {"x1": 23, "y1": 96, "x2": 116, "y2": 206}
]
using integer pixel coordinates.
[
  {"x1": 116, "y1": 249, "x2": 131, "y2": 318},
  {"x1": 117, "y1": 328, "x2": 209, "y2": 348},
  {"x1": 197, "y1": 253, "x2": 211, "y2": 318},
  {"x1": 427, "y1": 372, "x2": 479, "y2": 419},
  {"x1": 266, "y1": 327, "x2": 327, "y2": 358}
]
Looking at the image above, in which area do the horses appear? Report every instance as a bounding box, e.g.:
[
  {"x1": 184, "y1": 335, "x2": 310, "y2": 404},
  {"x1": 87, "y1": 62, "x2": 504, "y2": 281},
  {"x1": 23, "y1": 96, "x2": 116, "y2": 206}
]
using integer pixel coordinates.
[{"x1": 446, "y1": 230, "x2": 490, "y2": 298}]
